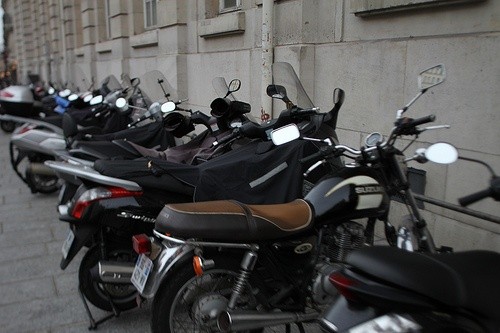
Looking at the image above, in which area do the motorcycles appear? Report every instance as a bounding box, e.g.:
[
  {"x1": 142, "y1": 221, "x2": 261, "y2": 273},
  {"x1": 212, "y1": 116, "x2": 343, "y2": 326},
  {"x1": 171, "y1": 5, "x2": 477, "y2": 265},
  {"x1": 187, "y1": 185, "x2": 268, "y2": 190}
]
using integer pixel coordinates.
[
  {"x1": 321, "y1": 143, "x2": 497, "y2": 333},
  {"x1": 44, "y1": 62, "x2": 346, "y2": 329},
  {"x1": 118, "y1": 78, "x2": 251, "y2": 165},
  {"x1": 131, "y1": 64, "x2": 453, "y2": 331},
  {"x1": 0, "y1": 74, "x2": 143, "y2": 191},
  {"x1": 39, "y1": 71, "x2": 193, "y2": 164}
]
